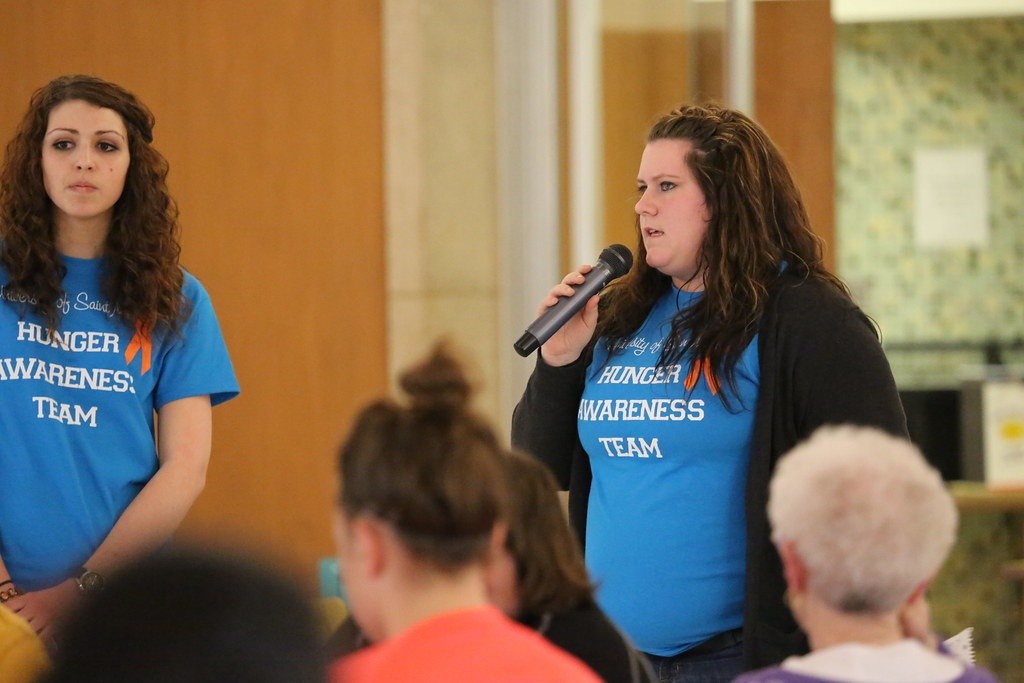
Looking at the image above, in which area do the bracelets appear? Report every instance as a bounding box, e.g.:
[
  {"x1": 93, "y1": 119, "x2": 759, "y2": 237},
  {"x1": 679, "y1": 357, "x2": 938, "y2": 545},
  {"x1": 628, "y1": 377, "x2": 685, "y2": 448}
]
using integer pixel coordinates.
[{"x1": 0, "y1": 580, "x2": 13, "y2": 588}]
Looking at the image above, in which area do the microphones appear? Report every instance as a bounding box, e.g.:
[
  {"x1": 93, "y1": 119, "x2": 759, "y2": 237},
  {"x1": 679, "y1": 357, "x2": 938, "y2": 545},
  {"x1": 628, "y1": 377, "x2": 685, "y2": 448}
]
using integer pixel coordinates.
[{"x1": 513, "y1": 244, "x2": 633, "y2": 357}]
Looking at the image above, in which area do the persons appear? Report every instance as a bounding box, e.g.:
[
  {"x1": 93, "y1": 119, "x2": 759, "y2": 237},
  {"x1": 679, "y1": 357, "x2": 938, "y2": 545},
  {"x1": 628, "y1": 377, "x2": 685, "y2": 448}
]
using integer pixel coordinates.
[
  {"x1": 510, "y1": 105, "x2": 912, "y2": 683},
  {"x1": 0, "y1": 73, "x2": 242, "y2": 661},
  {"x1": 728, "y1": 424, "x2": 998, "y2": 683},
  {"x1": 32, "y1": 338, "x2": 661, "y2": 683}
]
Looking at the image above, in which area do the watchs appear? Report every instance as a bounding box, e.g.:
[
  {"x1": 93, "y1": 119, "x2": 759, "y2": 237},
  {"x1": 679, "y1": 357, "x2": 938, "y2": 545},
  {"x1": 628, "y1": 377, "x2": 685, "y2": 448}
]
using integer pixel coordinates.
[{"x1": 75, "y1": 567, "x2": 104, "y2": 595}]
